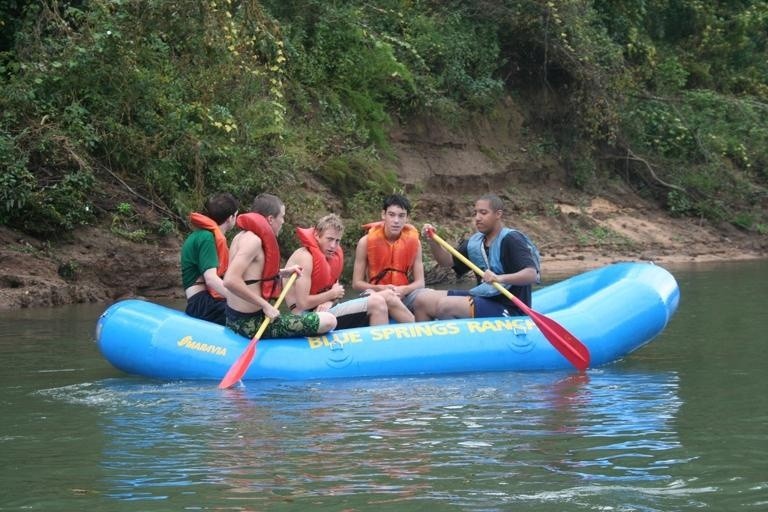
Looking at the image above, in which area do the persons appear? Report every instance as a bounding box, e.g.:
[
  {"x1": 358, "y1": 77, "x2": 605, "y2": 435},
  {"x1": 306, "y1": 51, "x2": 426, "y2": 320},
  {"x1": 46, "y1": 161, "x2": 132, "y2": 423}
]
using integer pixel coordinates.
[
  {"x1": 411, "y1": 192, "x2": 541, "y2": 323},
  {"x1": 219, "y1": 192, "x2": 338, "y2": 339},
  {"x1": 178, "y1": 190, "x2": 238, "y2": 326},
  {"x1": 351, "y1": 193, "x2": 435, "y2": 312},
  {"x1": 280, "y1": 210, "x2": 416, "y2": 329}
]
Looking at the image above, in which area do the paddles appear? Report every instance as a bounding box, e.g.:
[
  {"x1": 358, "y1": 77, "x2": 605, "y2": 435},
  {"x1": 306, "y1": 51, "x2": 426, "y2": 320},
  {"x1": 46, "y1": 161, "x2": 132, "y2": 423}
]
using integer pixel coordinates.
[
  {"x1": 427, "y1": 229, "x2": 590, "y2": 372},
  {"x1": 220, "y1": 268, "x2": 302, "y2": 388}
]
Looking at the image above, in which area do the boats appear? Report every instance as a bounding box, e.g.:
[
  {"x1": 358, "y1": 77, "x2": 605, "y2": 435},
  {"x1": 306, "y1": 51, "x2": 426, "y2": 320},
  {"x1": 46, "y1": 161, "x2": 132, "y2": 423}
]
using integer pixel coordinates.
[{"x1": 95, "y1": 263, "x2": 680, "y2": 380}]
